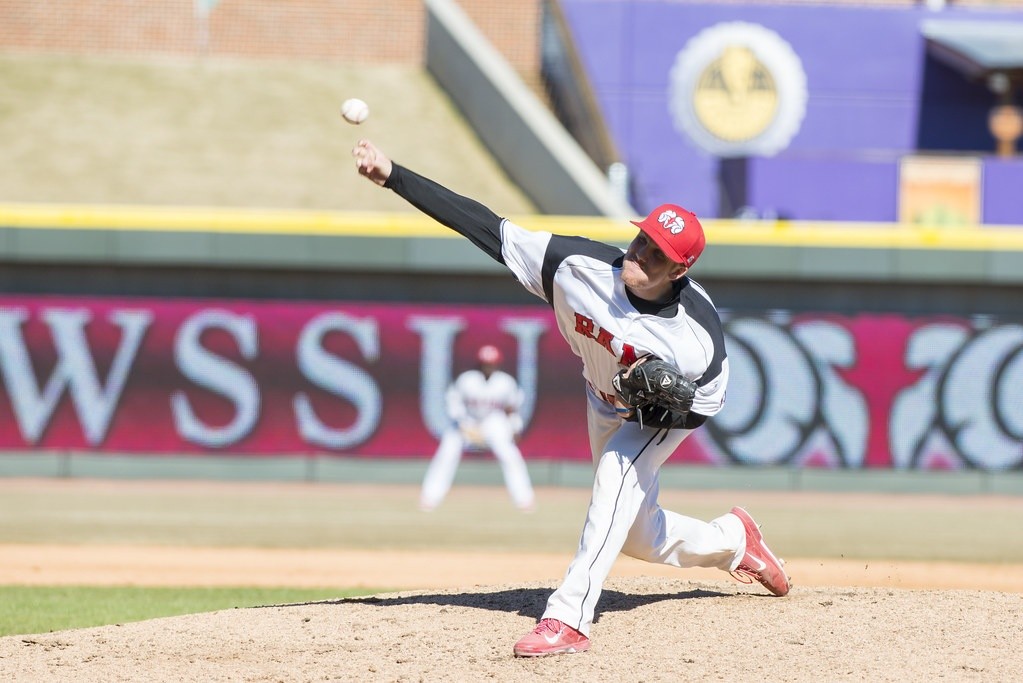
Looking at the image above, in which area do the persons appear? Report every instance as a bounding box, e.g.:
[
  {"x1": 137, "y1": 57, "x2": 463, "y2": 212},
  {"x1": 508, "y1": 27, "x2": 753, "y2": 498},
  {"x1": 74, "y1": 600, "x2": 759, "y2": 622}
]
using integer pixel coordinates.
[
  {"x1": 354, "y1": 140, "x2": 792, "y2": 657},
  {"x1": 420, "y1": 346, "x2": 536, "y2": 508}
]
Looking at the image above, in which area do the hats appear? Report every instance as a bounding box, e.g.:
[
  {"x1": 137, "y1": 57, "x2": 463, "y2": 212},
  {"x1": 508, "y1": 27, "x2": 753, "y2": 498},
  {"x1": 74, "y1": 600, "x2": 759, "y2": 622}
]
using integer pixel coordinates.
[
  {"x1": 476, "y1": 344, "x2": 504, "y2": 364},
  {"x1": 629, "y1": 204, "x2": 705, "y2": 270}
]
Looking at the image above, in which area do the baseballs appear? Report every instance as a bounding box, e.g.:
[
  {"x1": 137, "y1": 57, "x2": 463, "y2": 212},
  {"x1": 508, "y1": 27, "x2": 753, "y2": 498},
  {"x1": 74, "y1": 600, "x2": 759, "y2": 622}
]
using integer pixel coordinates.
[{"x1": 341, "y1": 98, "x2": 368, "y2": 125}]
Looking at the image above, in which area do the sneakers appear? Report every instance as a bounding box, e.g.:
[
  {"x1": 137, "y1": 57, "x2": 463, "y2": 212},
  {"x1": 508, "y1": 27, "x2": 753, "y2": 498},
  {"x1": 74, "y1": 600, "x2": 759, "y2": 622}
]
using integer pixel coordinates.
[
  {"x1": 513, "y1": 619, "x2": 590, "y2": 658},
  {"x1": 729, "y1": 506, "x2": 792, "y2": 597}
]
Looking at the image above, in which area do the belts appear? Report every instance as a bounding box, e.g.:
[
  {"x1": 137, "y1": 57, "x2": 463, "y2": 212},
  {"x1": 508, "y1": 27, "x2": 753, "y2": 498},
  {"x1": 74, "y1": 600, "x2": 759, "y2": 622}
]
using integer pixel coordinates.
[{"x1": 587, "y1": 382, "x2": 615, "y2": 407}]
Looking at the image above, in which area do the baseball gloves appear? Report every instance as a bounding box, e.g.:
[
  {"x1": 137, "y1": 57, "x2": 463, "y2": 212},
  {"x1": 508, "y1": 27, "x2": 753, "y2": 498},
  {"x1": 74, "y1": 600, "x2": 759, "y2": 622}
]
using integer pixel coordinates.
[{"x1": 611, "y1": 356, "x2": 697, "y2": 415}]
